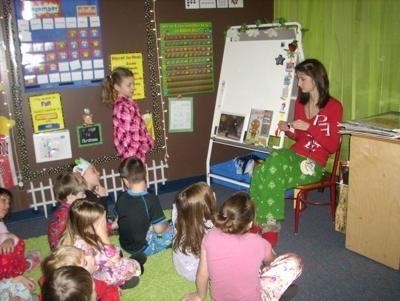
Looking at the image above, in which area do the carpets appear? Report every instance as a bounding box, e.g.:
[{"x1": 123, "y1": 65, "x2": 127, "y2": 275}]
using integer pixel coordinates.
[{"x1": 23, "y1": 209, "x2": 211, "y2": 301}]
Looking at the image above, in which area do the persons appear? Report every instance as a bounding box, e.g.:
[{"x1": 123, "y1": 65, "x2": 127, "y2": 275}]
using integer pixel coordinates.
[
  {"x1": 57, "y1": 198, "x2": 147, "y2": 289},
  {"x1": 115, "y1": 155, "x2": 174, "y2": 256},
  {"x1": 179, "y1": 190, "x2": 304, "y2": 301},
  {"x1": 38, "y1": 245, "x2": 120, "y2": 301},
  {"x1": 48, "y1": 171, "x2": 120, "y2": 252},
  {"x1": 68, "y1": 156, "x2": 109, "y2": 224},
  {"x1": 242, "y1": 59, "x2": 344, "y2": 248},
  {"x1": 0, "y1": 187, "x2": 40, "y2": 301},
  {"x1": 40, "y1": 266, "x2": 96, "y2": 301},
  {"x1": 173, "y1": 181, "x2": 216, "y2": 282},
  {"x1": 102, "y1": 67, "x2": 155, "y2": 192}
]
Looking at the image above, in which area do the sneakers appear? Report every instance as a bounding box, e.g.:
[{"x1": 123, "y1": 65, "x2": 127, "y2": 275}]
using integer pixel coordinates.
[
  {"x1": 244, "y1": 222, "x2": 281, "y2": 248},
  {"x1": 25, "y1": 251, "x2": 40, "y2": 272}
]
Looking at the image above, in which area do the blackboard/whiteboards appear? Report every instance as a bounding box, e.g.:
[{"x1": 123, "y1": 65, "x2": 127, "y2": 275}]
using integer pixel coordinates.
[{"x1": 209, "y1": 39, "x2": 298, "y2": 154}]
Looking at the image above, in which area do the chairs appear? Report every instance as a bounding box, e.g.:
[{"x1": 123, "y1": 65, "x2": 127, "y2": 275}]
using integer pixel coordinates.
[{"x1": 284, "y1": 136, "x2": 343, "y2": 236}]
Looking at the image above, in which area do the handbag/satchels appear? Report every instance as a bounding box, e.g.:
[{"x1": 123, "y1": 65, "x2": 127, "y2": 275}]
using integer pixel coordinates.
[{"x1": 300, "y1": 160, "x2": 315, "y2": 175}]
